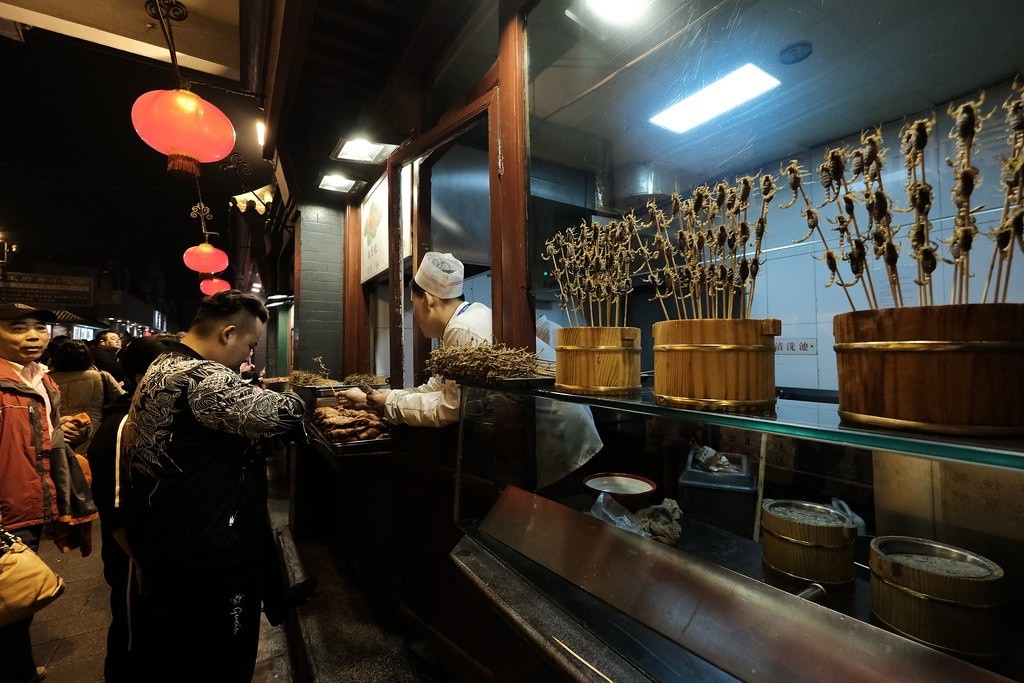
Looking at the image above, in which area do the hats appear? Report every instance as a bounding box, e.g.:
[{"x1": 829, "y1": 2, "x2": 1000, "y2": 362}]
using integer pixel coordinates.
[
  {"x1": 415, "y1": 251, "x2": 464, "y2": 299},
  {"x1": 1, "y1": 300, "x2": 58, "y2": 323}
]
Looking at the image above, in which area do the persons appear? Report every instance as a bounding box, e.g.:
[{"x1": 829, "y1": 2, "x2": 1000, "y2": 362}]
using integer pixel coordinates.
[
  {"x1": 335, "y1": 252, "x2": 603, "y2": 505},
  {"x1": 0, "y1": 288, "x2": 304, "y2": 683}
]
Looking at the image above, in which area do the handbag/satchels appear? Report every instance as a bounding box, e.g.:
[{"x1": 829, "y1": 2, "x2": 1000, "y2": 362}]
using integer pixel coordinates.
[{"x1": 0, "y1": 528, "x2": 66, "y2": 631}]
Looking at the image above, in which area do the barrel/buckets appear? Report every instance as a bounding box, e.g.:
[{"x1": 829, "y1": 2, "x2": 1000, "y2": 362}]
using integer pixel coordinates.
[
  {"x1": 869, "y1": 534, "x2": 1006, "y2": 658},
  {"x1": 831, "y1": 303, "x2": 1024, "y2": 438},
  {"x1": 646, "y1": 319, "x2": 783, "y2": 414},
  {"x1": 552, "y1": 325, "x2": 642, "y2": 398},
  {"x1": 758, "y1": 501, "x2": 860, "y2": 593}
]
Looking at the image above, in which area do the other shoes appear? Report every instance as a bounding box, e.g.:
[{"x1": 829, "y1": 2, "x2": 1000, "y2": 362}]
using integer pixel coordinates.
[{"x1": 26, "y1": 666, "x2": 48, "y2": 683}]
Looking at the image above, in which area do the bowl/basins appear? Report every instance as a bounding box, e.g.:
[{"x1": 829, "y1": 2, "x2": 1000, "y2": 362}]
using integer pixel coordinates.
[{"x1": 583, "y1": 472, "x2": 658, "y2": 511}]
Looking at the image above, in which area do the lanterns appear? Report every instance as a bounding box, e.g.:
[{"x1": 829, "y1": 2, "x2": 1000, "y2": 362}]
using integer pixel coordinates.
[
  {"x1": 183, "y1": 243, "x2": 228, "y2": 281},
  {"x1": 131, "y1": 89, "x2": 236, "y2": 177},
  {"x1": 200, "y1": 278, "x2": 230, "y2": 296}
]
